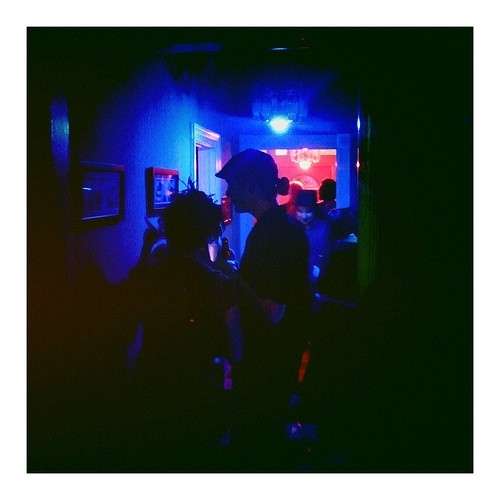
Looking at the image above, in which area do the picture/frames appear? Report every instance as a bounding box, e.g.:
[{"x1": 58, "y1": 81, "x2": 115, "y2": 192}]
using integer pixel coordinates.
[
  {"x1": 146, "y1": 168, "x2": 179, "y2": 217},
  {"x1": 221, "y1": 196, "x2": 232, "y2": 227},
  {"x1": 75, "y1": 160, "x2": 125, "y2": 230}
]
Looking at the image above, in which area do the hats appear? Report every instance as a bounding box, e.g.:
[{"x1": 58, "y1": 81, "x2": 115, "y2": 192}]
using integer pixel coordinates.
[
  {"x1": 214, "y1": 148, "x2": 280, "y2": 182},
  {"x1": 291, "y1": 190, "x2": 317, "y2": 208}
]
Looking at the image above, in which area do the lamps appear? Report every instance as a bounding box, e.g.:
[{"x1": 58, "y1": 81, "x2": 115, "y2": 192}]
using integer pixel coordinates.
[
  {"x1": 250, "y1": 82, "x2": 305, "y2": 135},
  {"x1": 289, "y1": 149, "x2": 320, "y2": 171}
]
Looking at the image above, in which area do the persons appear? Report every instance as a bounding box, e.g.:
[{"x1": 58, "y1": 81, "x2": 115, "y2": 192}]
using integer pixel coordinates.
[
  {"x1": 108, "y1": 190, "x2": 228, "y2": 442},
  {"x1": 293, "y1": 189, "x2": 331, "y2": 280},
  {"x1": 215, "y1": 152, "x2": 313, "y2": 379},
  {"x1": 316, "y1": 179, "x2": 337, "y2": 218}
]
